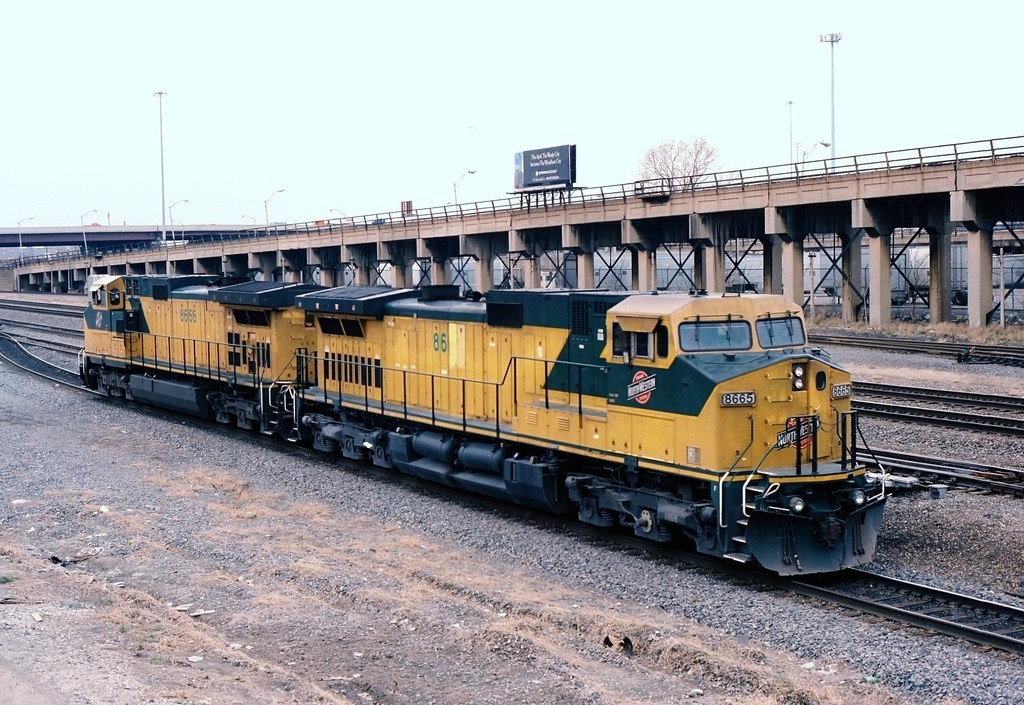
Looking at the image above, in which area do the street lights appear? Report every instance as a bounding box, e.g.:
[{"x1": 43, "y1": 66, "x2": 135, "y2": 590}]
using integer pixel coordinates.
[
  {"x1": 821, "y1": 31, "x2": 845, "y2": 173},
  {"x1": 154, "y1": 91, "x2": 168, "y2": 242},
  {"x1": 264, "y1": 188, "x2": 285, "y2": 230},
  {"x1": 80, "y1": 210, "x2": 98, "y2": 256},
  {"x1": 454, "y1": 170, "x2": 476, "y2": 213},
  {"x1": 801, "y1": 141, "x2": 831, "y2": 175},
  {"x1": 18, "y1": 218, "x2": 33, "y2": 264},
  {"x1": 169, "y1": 200, "x2": 189, "y2": 244}
]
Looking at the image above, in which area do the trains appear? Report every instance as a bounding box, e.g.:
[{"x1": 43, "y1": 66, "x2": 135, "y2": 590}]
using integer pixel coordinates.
[{"x1": 79, "y1": 262, "x2": 891, "y2": 583}]
